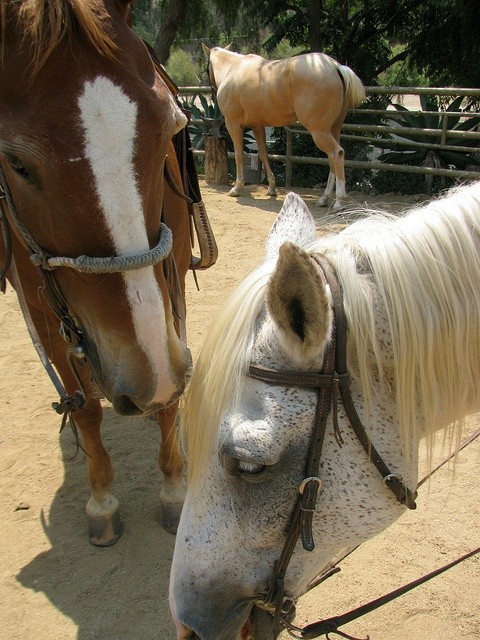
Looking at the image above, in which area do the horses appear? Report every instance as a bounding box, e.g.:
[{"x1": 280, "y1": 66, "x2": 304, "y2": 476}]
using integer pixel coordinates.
[
  {"x1": 196, "y1": 36, "x2": 372, "y2": 207},
  {"x1": 165, "y1": 184, "x2": 480, "y2": 632},
  {"x1": 1, "y1": 0, "x2": 223, "y2": 551}
]
